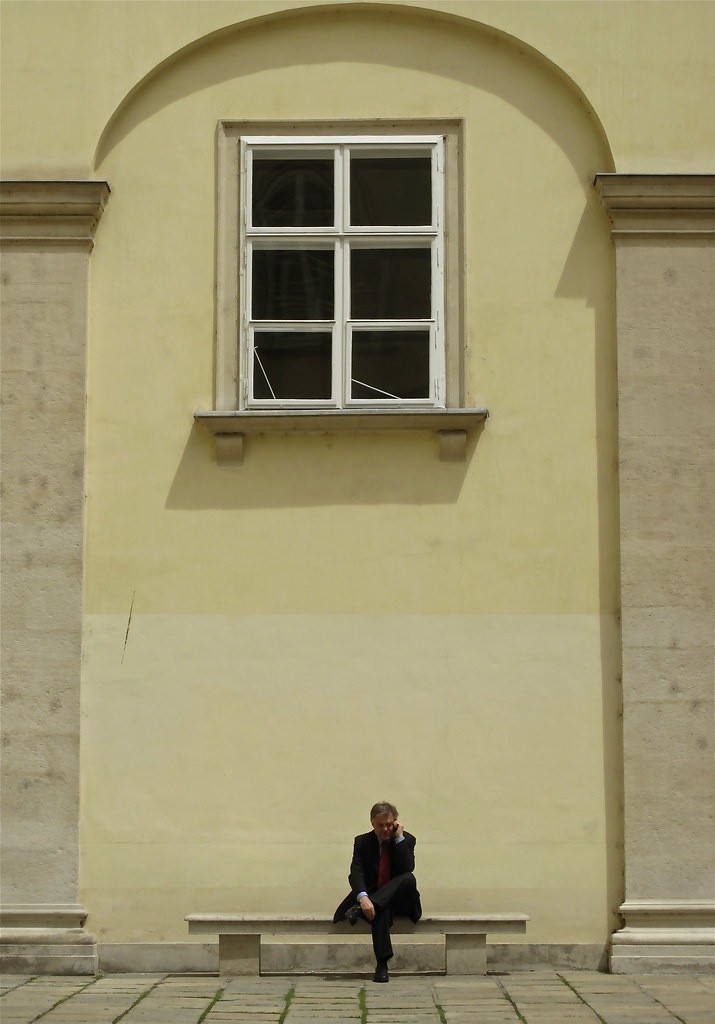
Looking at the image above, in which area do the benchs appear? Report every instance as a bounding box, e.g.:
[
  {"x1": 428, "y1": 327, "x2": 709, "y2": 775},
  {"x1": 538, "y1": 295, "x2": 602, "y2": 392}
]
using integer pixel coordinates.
[{"x1": 184, "y1": 910, "x2": 529, "y2": 977}]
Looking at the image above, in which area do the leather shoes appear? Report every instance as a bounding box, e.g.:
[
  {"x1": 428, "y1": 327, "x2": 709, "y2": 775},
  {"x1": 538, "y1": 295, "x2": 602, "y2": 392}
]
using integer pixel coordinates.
[
  {"x1": 345, "y1": 904, "x2": 360, "y2": 926},
  {"x1": 373, "y1": 967, "x2": 389, "y2": 982}
]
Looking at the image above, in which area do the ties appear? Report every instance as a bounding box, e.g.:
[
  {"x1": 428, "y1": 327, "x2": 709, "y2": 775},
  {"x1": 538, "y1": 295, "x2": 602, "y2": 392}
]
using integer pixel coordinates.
[{"x1": 378, "y1": 840, "x2": 390, "y2": 889}]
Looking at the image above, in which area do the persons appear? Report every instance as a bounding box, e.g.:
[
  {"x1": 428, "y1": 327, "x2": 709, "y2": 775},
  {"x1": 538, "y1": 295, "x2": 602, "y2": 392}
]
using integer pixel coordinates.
[{"x1": 334, "y1": 802, "x2": 422, "y2": 983}]
